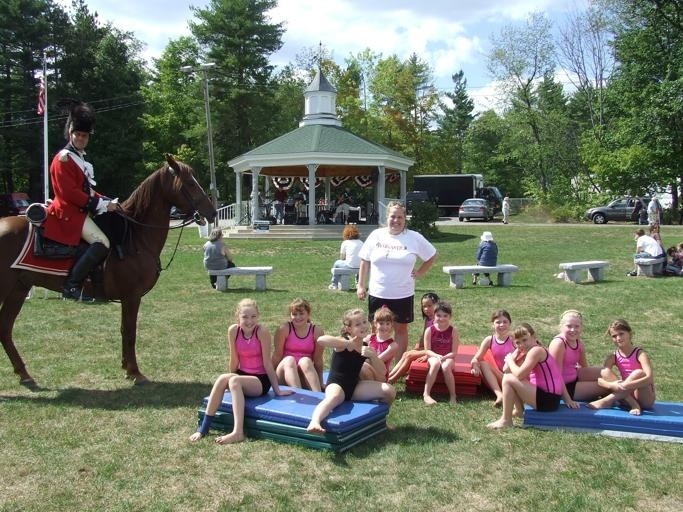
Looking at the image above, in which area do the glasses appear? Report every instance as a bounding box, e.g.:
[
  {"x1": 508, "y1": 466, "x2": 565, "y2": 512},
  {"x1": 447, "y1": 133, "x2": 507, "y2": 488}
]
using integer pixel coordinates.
[{"x1": 389, "y1": 201, "x2": 405, "y2": 207}]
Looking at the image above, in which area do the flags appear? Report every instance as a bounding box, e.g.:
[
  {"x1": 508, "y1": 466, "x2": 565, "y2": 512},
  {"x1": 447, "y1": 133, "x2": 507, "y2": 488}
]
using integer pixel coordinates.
[{"x1": 36, "y1": 59, "x2": 46, "y2": 115}]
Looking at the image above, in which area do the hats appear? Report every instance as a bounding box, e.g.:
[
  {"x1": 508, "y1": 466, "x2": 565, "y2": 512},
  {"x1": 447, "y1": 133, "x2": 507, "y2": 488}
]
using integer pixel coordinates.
[
  {"x1": 69, "y1": 101, "x2": 95, "y2": 135},
  {"x1": 481, "y1": 232, "x2": 493, "y2": 241}
]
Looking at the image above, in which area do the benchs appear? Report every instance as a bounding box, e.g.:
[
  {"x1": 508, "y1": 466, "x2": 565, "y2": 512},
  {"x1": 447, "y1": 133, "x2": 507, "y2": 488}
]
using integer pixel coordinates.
[
  {"x1": 634, "y1": 256, "x2": 667, "y2": 277},
  {"x1": 558, "y1": 261, "x2": 609, "y2": 284},
  {"x1": 330, "y1": 267, "x2": 360, "y2": 291},
  {"x1": 208, "y1": 267, "x2": 274, "y2": 291},
  {"x1": 442, "y1": 264, "x2": 518, "y2": 288}
]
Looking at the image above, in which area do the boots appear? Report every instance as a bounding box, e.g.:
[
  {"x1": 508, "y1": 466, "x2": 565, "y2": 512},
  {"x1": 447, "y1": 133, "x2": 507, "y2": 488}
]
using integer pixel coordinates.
[{"x1": 62, "y1": 242, "x2": 109, "y2": 300}]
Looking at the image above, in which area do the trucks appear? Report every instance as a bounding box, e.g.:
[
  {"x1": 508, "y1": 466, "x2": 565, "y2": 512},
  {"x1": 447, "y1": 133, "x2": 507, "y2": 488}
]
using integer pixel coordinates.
[{"x1": 412, "y1": 174, "x2": 502, "y2": 217}]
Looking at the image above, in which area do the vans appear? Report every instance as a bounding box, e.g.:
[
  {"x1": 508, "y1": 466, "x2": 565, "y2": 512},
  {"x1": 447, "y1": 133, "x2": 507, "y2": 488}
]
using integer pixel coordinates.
[{"x1": 0, "y1": 193, "x2": 34, "y2": 218}]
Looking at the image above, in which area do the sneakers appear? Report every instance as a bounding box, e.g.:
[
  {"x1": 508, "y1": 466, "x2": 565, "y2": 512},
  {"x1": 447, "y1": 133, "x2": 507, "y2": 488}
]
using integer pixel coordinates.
[
  {"x1": 329, "y1": 284, "x2": 337, "y2": 289},
  {"x1": 626, "y1": 272, "x2": 637, "y2": 276}
]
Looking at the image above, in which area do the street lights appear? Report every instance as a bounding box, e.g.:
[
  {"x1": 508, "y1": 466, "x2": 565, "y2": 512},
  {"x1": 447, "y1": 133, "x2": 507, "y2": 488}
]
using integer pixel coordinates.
[{"x1": 180, "y1": 62, "x2": 217, "y2": 208}]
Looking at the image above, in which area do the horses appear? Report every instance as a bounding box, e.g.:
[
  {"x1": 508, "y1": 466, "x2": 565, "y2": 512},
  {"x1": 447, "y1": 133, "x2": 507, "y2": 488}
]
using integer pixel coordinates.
[{"x1": 0, "y1": 152, "x2": 218, "y2": 386}]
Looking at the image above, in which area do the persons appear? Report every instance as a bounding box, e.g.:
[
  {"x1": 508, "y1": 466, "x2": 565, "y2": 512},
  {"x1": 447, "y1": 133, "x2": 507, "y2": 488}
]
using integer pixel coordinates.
[
  {"x1": 47, "y1": 104, "x2": 120, "y2": 305},
  {"x1": 501, "y1": 197, "x2": 510, "y2": 225},
  {"x1": 386, "y1": 293, "x2": 441, "y2": 384},
  {"x1": 549, "y1": 307, "x2": 618, "y2": 409},
  {"x1": 485, "y1": 322, "x2": 563, "y2": 429},
  {"x1": 271, "y1": 297, "x2": 326, "y2": 393},
  {"x1": 305, "y1": 308, "x2": 396, "y2": 434},
  {"x1": 626, "y1": 196, "x2": 683, "y2": 277},
  {"x1": 327, "y1": 225, "x2": 364, "y2": 290},
  {"x1": 188, "y1": 298, "x2": 296, "y2": 445},
  {"x1": 356, "y1": 199, "x2": 438, "y2": 376},
  {"x1": 586, "y1": 318, "x2": 656, "y2": 416},
  {"x1": 250, "y1": 179, "x2": 378, "y2": 224},
  {"x1": 359, "y1": 304, "x2": 400, "y2": 383},
  {"x1": 472, "y1": 231, "x2": 498, "y2": 286},
  {"x1": 422, "y1": 301, "x2": 459, "y2": 406},
  {"x1": 203, "y1": 226, "x2": 235, "y2": 289},
  {"x1": 471, "y1": 308, "x2": 518, "y2": 407}
]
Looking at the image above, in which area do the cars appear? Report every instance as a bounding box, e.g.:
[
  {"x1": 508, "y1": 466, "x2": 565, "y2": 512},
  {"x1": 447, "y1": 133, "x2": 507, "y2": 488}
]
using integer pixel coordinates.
[
  {"x1": 584, "y1": 197, "x2": 661, "y2": 224},
  {"x1": 457, "y1": 197, "x2": 493, "y2": 222},
  {"x1": 405, "y1": 191, "x2": 437, "y2": 215}
]
